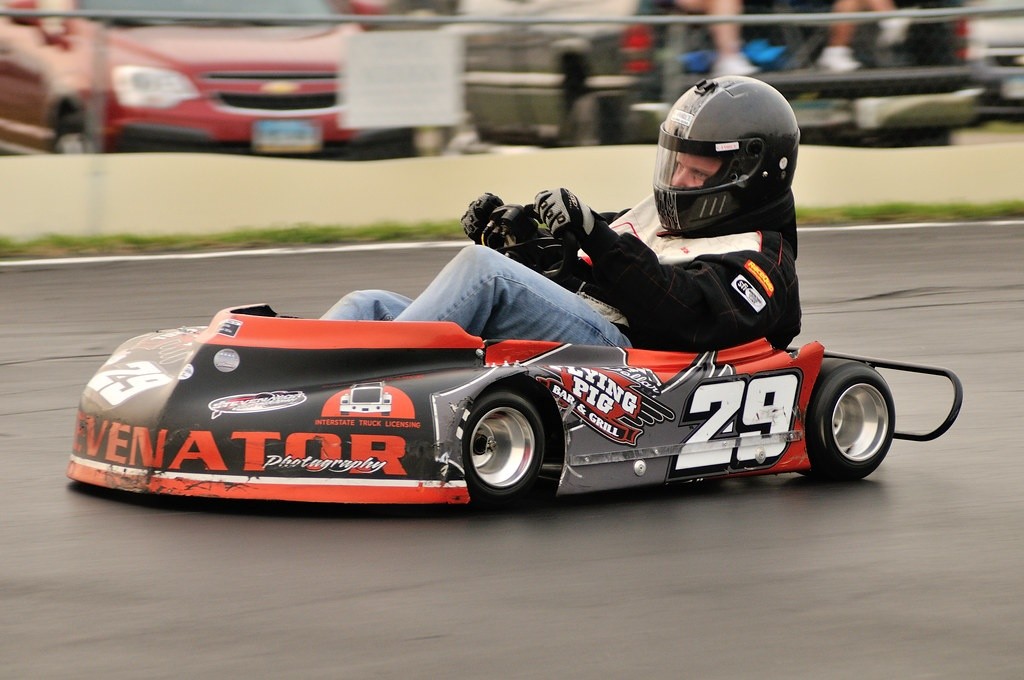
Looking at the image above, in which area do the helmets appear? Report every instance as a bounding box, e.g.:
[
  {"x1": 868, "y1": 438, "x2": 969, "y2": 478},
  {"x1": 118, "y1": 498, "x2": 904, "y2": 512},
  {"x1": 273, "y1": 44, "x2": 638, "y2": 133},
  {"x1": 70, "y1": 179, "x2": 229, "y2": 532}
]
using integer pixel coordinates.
[{"x1": 652, "y1": 76, "x2": 800, "y2": 231}]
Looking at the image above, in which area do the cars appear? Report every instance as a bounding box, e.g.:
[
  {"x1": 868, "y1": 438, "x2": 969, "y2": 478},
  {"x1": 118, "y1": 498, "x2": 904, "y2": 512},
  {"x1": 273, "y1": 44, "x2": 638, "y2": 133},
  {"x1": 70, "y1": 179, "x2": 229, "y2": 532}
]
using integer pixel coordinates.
[
  {"x1": 458, "y1": 0, "x2": 981, "y2": 146},
  {"x1": 0, "y1": 0, "x2": 450, "y2": 163}
]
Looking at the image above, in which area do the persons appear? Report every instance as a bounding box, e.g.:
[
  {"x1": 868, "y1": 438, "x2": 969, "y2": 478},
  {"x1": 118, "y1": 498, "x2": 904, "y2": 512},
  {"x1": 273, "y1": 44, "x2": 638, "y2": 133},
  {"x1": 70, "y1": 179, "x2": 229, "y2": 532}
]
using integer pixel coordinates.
[
  {"x1": 619, "y1": 0, "x2": 927, "y2": 81},
  {"x1": 318, "y1": 74, "x2": 805, "y2": 355}
]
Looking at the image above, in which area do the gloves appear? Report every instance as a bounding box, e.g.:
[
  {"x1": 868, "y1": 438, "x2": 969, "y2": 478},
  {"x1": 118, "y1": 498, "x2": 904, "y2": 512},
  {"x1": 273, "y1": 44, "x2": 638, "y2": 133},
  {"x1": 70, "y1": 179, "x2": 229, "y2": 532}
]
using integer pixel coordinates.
[
  {"x1": 460, "y1": 192, "x2": 502, "y2": 243},
  {"x1": 533, "y1": 188, "x2": 598, "y2": 244}
]
